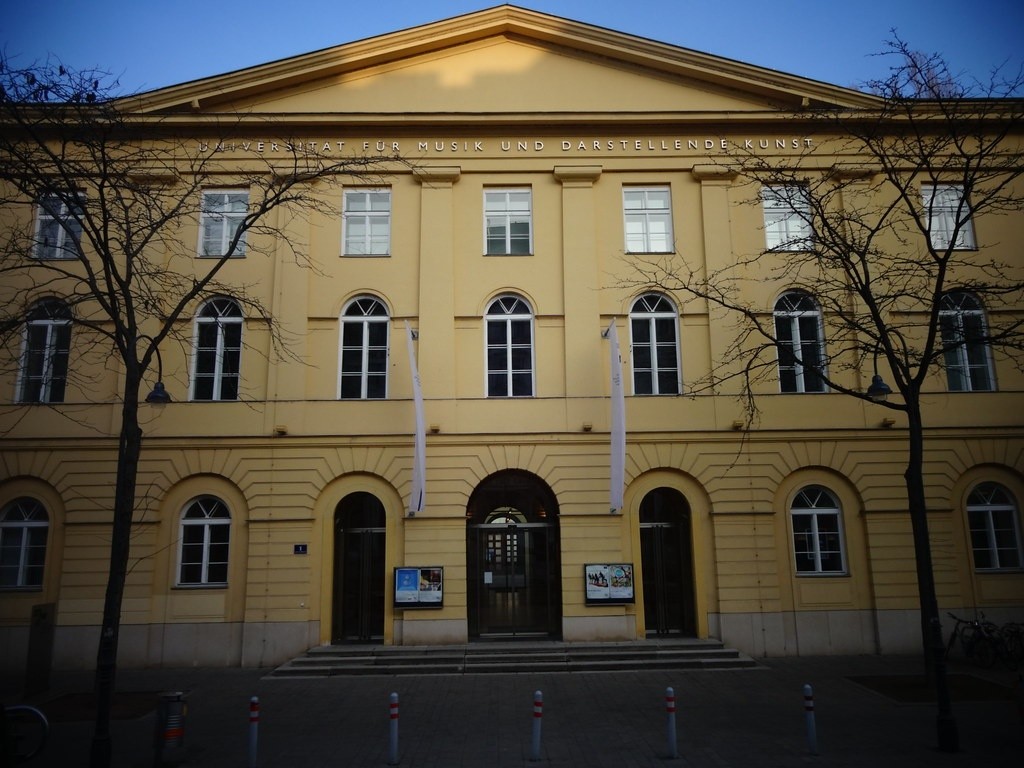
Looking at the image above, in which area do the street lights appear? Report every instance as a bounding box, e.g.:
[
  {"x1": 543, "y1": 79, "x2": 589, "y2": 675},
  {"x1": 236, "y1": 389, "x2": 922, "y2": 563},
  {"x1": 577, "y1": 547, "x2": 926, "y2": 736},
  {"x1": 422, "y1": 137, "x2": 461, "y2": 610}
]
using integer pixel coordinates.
[
  {"x1": 86, "y1": 335, "x2": 173, "y2": 768},
  {"x1": 866, "y1": 326, "x2": 963, "y2": 754}
]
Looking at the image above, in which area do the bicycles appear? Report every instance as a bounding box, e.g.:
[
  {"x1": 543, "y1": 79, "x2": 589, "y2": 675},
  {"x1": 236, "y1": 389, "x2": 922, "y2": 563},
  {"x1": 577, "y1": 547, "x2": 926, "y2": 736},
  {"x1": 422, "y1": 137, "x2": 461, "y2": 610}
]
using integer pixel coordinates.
[{"x1": 947, "y1": 611, "x2": 1024, "y2": 669}]
